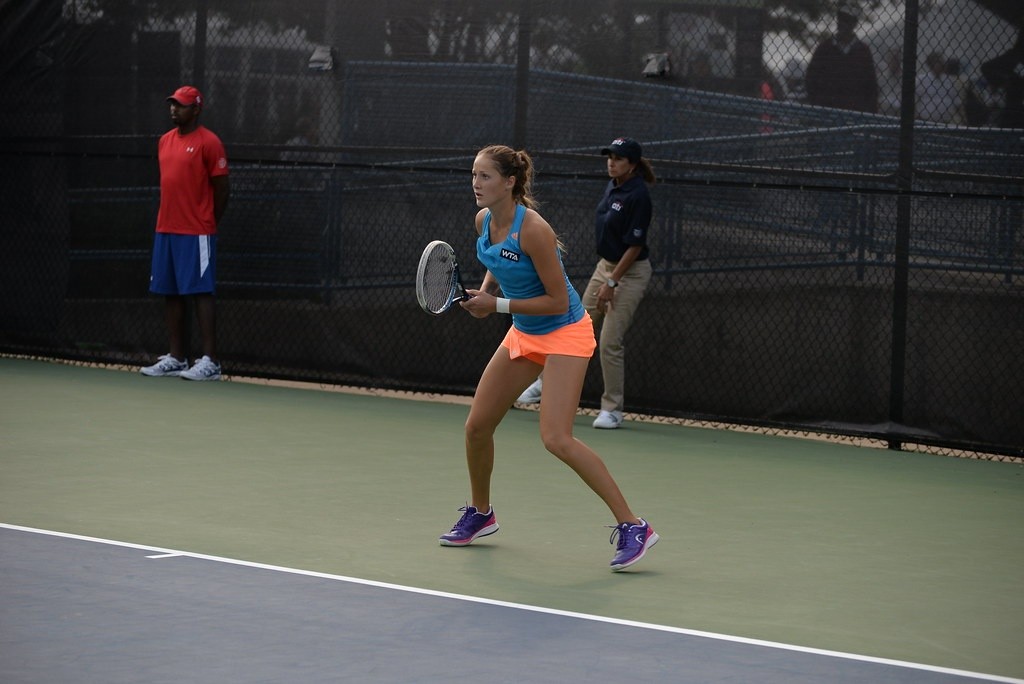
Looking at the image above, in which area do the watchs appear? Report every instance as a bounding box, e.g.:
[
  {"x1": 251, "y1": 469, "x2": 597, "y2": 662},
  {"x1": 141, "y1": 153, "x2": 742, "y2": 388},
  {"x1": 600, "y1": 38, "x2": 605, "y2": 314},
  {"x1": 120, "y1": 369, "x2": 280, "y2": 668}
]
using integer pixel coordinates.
[{"x1": 607, "y1": 278, "x2": 618, "y2": 288}]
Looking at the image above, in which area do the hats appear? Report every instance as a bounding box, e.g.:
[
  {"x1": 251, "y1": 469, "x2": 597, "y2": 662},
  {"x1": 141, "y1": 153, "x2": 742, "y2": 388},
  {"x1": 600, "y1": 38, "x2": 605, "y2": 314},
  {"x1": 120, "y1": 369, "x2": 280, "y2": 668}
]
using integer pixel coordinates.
[
  {"x1": 166, "y1": 86, "x2": 204, "y2": 107},
  {"x1": 600, "y1": 137, "x2": 642, "y2": 163}
]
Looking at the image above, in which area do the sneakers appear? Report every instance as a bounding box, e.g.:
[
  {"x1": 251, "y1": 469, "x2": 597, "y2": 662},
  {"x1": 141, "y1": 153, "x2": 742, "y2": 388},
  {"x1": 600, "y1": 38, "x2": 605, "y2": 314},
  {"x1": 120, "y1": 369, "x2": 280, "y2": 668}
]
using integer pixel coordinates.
[
  {"x1": 592, "y1": 410, "x2": 623, "y2": 428},
  {"x1": 438, "y1": 501, "x2": 500, "y2": 546},
  {"x1": 179, "y1": 355, "x2": 221, "y2": 381},
  {"x1": 140, "y1": 353, "x2": 188, "y2": 378},
  {"x1": 516, "y1": 379, "x2": 542, "y2": 404},
  {"x1": 603, "y1": 517, "x2": 659, "y2": 571}
]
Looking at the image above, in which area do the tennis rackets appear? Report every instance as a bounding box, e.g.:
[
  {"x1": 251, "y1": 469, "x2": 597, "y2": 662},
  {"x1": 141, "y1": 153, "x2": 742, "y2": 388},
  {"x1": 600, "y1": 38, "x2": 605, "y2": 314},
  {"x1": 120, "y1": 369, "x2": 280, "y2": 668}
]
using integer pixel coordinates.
[{"x1": 416, "y1": 241, "x2": 474, "y2": 317}]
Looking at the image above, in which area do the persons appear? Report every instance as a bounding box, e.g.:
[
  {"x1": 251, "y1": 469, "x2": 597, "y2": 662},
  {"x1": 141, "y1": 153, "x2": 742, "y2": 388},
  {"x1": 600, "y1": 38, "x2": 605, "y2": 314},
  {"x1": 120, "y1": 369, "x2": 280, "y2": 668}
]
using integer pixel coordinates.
[
  {"x1": 140, "y1": 86, "x2": 229, "y2": 381},
  {"x1": 424, "y1": 0, "x2": 1022, "y2": 131},
  {"x1": 440, "y1": 139, "x2": 660, "y2": 572}
]
